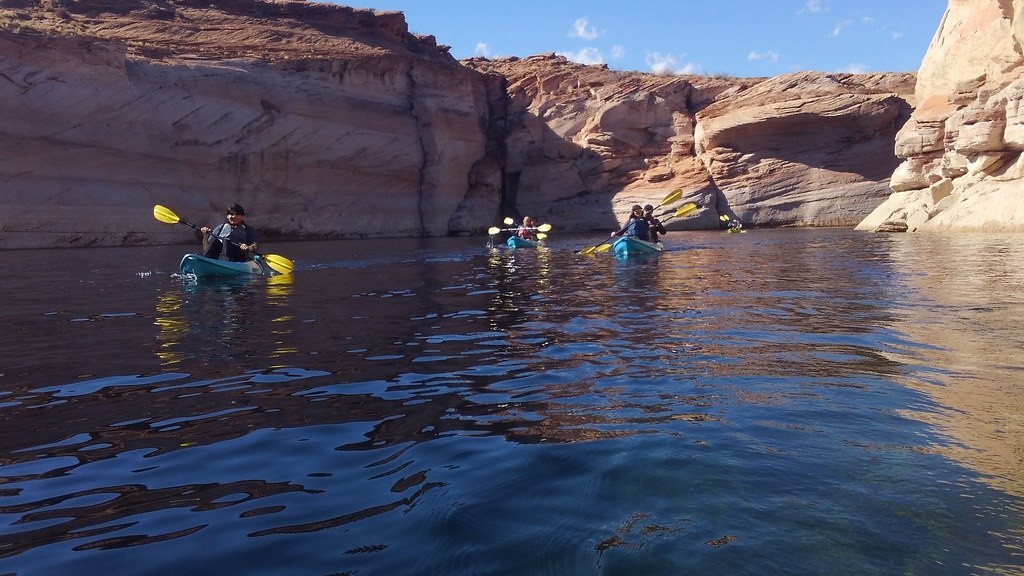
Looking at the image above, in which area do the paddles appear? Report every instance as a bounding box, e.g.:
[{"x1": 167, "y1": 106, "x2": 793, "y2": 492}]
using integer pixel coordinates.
[
  {"x1": 580, "y1": 188, "x2": 683, "y2": 255},
  {"x1": 719, "y1": 215, "x2": 744, "y2": 233},
  {"x1": 503, "y1": 216, "x2": 548, "y2": 241},
  {"x1": 594, "y1": 202, "x2": 697, "y2": 252},
  {"x1": 724, "y1": 214, "x2": 746, "y2": 233},
  {"x1": 152, "y1": 203, "x2": 295, "y2": 274},
  {"x1": 488, "y1": 223, "x2": 552, "y2": 235}
]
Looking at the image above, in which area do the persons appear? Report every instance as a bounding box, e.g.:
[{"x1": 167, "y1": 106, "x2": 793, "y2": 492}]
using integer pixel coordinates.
[
  {"x1": 611, "y1": 205, "x2": 666, "y2": 242},
  {"x1": 510, "y1": 216, "x2": 538, "y2": 240},
  {"x1": 201, "y1": 204, "x2": 259, "y2": 262},
  {"x1": 728, "y1": 220, "x2": 742, "y2": 230}
]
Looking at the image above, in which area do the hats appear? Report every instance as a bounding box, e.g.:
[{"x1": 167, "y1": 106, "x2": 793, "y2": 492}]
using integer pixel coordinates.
[
  {"x1": 644, "y1": 205, "x2": 653, "y2": 213},
  {"x1": 632, "y1": 205, "x2": 642, "y2": 211}
]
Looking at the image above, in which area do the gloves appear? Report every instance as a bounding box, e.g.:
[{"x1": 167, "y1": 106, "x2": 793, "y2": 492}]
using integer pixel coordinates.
[{"x1": 651, "y1": 219, "x2": 659, "y2": 226}]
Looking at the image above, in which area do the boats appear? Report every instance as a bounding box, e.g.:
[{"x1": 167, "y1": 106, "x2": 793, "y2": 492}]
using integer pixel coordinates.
[
  {"x1": 728, "y1": 228, "x2": 738, "y2": 234},
  {"x1": 612, "y1": 234, "x2": 659, "y2": 252},
  {"x1": 181, "y1": 276, "x2": 258, "y2": 294},
  {"x1": 617, "y1": 253, "x2": 651, "y2": 268},
  {"x1": 178, "y1": 254, "x2": 267, "y2": 276},
  {"x1": 506, "y1": 236, "x2": 534, "y2": 249}
]
